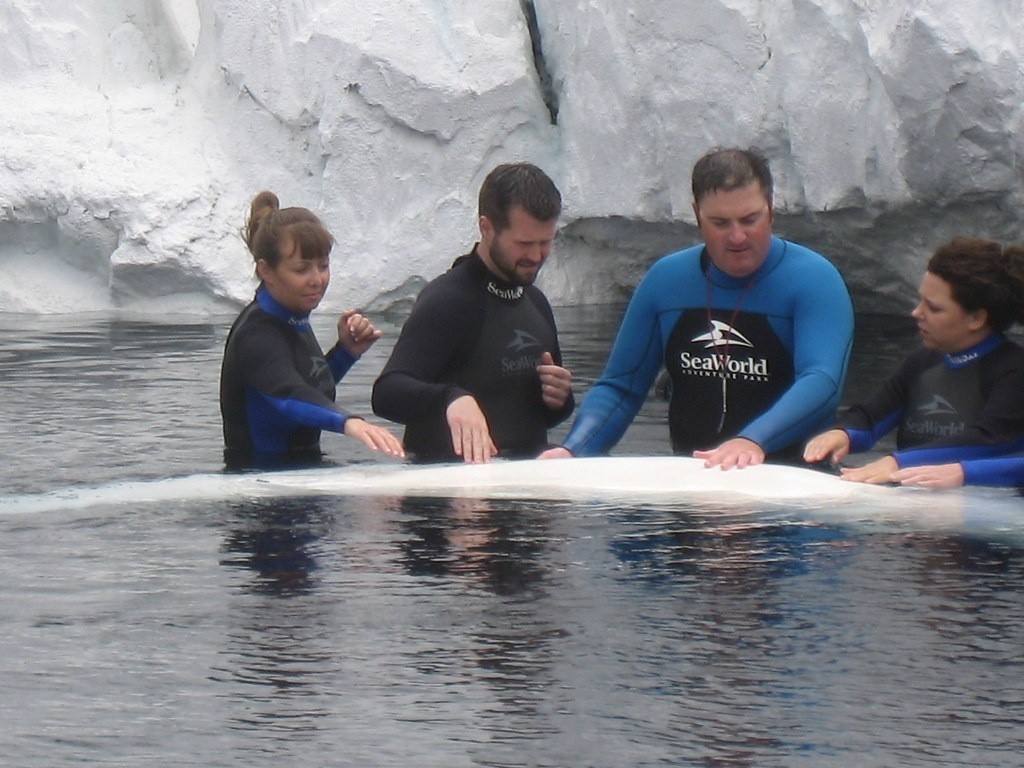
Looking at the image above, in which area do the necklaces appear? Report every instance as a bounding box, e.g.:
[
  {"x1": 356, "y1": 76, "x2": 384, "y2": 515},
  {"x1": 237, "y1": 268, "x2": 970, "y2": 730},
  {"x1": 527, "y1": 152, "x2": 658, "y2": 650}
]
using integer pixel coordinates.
[{"x1": 704, "y1": 258, "x2": 754, "y2": 435}]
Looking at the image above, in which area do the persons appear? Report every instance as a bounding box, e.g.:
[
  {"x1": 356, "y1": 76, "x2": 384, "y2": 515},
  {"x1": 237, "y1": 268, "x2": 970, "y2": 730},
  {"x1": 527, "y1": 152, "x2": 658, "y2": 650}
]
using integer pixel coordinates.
[
  {"x1": 371, "y1": 161, "x2": 576, "y2": 466},
  {"x1": 220, "y1": 192, "x2": 405, "y2": 468},
  {"x1": 890, "y1": 451, "x2": 1024, "y2": 487},
  {"x1": 536, "y1": 147, "x2": 855, "y2": 471},
  {"x1": 804, "y1": 235, "x2": 1024, "y2": 483}
]
